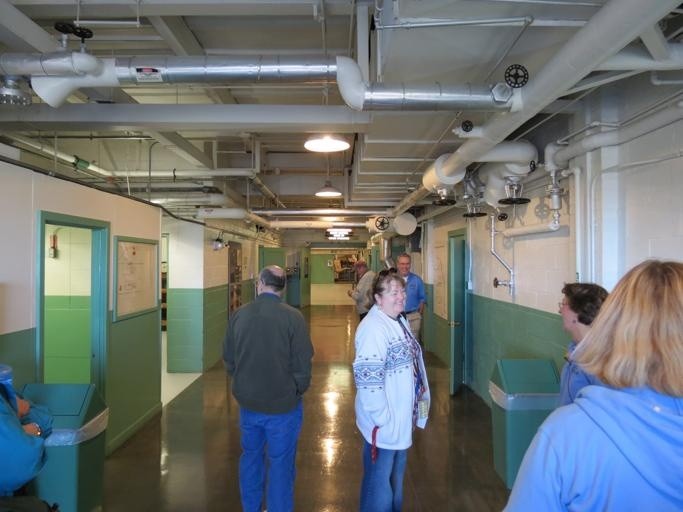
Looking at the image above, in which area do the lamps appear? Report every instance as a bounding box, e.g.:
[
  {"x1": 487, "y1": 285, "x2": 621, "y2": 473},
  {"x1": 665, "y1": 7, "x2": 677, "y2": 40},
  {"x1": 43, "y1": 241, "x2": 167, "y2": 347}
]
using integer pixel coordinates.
[
  {"x1": 213, "y1": 236, "x2": 230, "y2": 250},
  {"x1": 315, "y1": 90, "x2": 342, "y2": 197},
  {"x1": 304, "y1": 1, "x2": 350, "y2": 153}
]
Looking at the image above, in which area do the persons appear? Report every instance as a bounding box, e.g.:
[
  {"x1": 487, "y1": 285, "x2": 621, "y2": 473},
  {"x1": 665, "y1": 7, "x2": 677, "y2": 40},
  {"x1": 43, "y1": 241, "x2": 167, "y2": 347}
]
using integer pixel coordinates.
[
  {"x1": 220, "y1": 263, "x2": 315, "y2": 512},
  {"x1": 345, "y1": 258, "x2": 378, "y2": 320},
  {"x1": 0, "y1": 362, "x2": 57, "y2": 511},
  {"x1": 555, "y1": 282, "x2": 611, "y2": 404},
  {"x1": 396, "y1": 252, "x2": 427, "y2": 348},
  {"x1": 344, "y1": 267, "x2": 432, "y2": 512},
  {"x1": 497, "y1": 253, "x2": 681, "y2": 512}
]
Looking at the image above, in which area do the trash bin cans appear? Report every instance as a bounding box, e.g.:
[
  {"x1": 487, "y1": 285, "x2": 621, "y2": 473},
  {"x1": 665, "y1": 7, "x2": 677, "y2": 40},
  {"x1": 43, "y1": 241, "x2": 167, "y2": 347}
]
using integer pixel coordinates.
[
  {"x1": 19, "y1": 383, "x2": 109, "y2": 512},
  {"x1": 488, "y1": 359, "x2": 561, "y2": 489}
]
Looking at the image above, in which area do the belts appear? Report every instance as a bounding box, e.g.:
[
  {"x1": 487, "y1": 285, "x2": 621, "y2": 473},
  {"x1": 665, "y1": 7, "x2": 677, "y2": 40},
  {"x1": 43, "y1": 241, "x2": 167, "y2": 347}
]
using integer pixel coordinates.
[{"x1": 405, "y1": 309, "x2": 415, "y2": 314}]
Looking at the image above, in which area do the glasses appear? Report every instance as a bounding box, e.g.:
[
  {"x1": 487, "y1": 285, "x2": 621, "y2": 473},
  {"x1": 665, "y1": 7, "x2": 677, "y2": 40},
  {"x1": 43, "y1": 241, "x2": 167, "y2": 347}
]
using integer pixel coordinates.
[
  {"x1": 558, "y1": 302, "x2": 569, "y2": 309},
  {"x1": 254, "y1": 279, "x2": 261, "y2": 287},
  {"x1": 379, "y1": 266, "x2": 398, "y2": 277}
]
